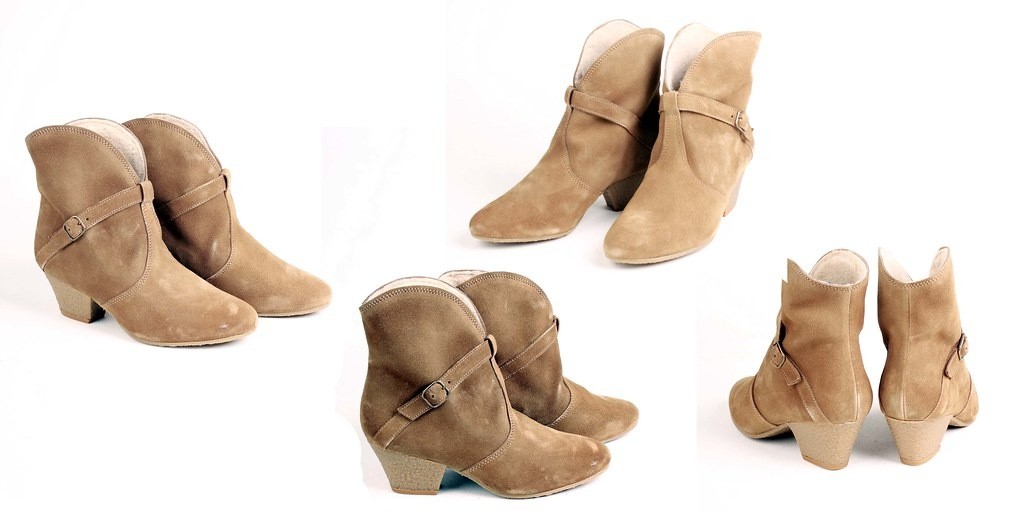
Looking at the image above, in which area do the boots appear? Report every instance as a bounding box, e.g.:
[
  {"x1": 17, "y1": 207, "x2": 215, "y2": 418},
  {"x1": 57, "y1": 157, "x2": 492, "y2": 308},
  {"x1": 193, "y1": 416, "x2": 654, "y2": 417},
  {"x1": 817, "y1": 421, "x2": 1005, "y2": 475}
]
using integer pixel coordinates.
[
  {"x1": 121, "y1": 113, "x2": 331, "y2": 321},
  {"x1": 468, "y1": 19, "x2": 665, "y2": 246},
  {"x1": 729, "y1": 248, "x2": 873, "y2": 470},
  {"x1": 436, "y1": 268, "x2": 640, "y2": 444},
  {"x1": 603, "y1": 22, "x2": 761, "y2": 267},
  {"x1": 359, "y1": 276, "x2": 612, "y2": 501},
  {"x1": 24, "y1": 118, "x2": 257, "y2": 349},
  {"x1": 878, "y1": 245, "x2": 979, "y2": 466}
]
